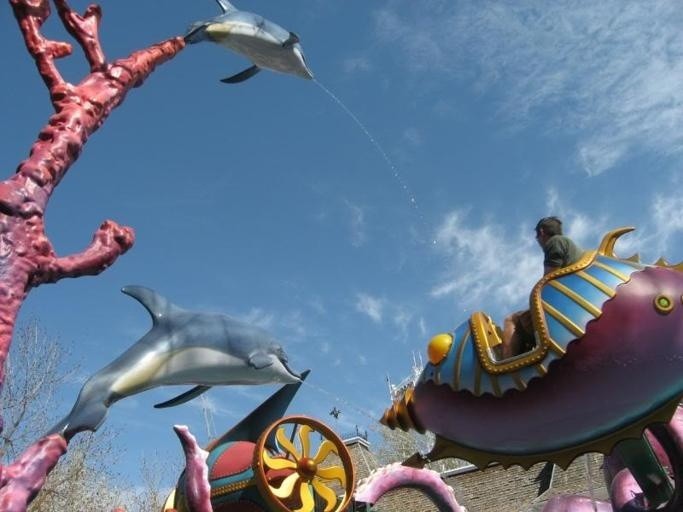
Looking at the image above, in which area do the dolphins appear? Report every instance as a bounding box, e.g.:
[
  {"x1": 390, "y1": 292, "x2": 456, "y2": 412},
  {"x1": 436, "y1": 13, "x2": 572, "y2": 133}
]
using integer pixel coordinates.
[
  {"x1": 184, "y1": 0, "x2": 314, "y2": 84},
  {"x1": 45, "y1": 284, "x2": 303, "y2": 447}
]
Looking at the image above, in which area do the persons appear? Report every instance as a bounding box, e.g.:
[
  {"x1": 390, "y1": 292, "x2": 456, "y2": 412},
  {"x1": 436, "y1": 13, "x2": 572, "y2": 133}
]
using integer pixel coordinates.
[{"x1": 501, "y1": 215, "x2": 584, "y2": 359}]
